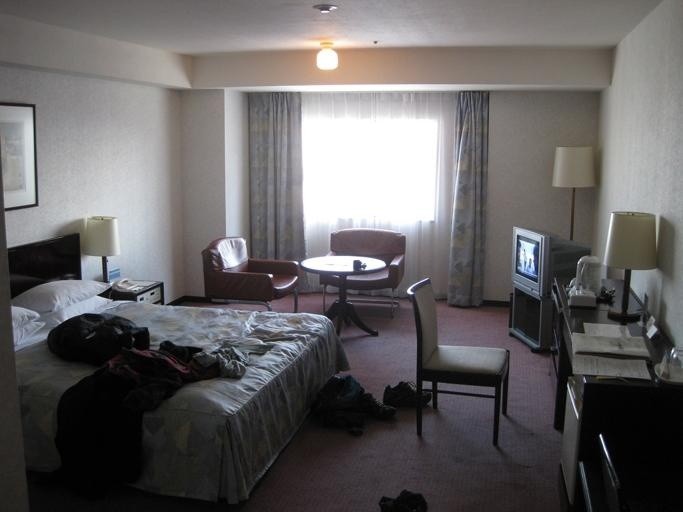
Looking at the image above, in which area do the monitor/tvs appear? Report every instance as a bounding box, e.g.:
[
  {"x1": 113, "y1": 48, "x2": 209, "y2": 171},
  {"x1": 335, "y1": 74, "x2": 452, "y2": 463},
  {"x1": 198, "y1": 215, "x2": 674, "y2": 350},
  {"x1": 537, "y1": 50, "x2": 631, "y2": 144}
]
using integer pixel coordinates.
[{"x1": 511, "y1": 226, "x2": 592, "y2": 297}]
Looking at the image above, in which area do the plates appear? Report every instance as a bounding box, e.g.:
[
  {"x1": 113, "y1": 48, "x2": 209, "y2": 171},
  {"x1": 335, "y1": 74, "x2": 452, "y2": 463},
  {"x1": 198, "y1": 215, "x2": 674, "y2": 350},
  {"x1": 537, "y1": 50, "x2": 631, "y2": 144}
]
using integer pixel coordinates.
[{"x1": 654, "y1": 362, "x2": 682, "y2": 385}]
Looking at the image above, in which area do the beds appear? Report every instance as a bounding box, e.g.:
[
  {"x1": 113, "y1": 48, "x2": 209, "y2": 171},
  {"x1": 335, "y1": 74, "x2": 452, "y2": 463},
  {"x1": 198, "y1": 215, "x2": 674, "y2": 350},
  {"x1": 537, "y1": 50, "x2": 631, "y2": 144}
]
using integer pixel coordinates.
[{"x1": 6, "y1": 232, "x2": 354, "y2": 502}]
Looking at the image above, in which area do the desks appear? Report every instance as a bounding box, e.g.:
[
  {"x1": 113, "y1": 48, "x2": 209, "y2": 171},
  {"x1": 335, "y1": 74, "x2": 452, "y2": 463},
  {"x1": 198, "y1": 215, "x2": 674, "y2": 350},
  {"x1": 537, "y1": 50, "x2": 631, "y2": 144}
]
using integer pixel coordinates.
[{"x1": 300, "y1": 255, "x2": 388, "y2": 343}]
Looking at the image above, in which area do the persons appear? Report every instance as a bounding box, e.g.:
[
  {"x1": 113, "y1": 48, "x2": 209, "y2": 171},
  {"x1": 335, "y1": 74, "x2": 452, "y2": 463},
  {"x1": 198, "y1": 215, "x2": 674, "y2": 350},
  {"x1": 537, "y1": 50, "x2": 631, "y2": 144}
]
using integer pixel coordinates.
[
  {"x1": 526, "y1": 259, "x2": 533, "y2": 274},
  {"x1": 522, "y1": 249, "x2": 527, "y2": 271}
]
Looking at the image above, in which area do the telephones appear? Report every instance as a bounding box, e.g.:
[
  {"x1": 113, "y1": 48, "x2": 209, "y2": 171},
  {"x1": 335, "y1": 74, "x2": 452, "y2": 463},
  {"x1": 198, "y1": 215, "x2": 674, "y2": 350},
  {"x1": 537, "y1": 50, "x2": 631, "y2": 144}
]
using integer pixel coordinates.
[{"x1": 113, "y1": 278, "x2": 137, "y2": 291}]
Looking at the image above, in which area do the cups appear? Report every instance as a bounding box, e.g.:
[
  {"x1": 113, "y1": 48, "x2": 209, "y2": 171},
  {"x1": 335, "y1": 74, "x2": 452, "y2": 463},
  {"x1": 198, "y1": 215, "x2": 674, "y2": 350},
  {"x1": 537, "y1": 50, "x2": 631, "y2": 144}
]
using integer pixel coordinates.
[
  {"x1": 352, "y1": 260, "x2": 360, "y2": 272},
  {"x1": 670, "y1": 346, "x2": 682, "y2": 378}
]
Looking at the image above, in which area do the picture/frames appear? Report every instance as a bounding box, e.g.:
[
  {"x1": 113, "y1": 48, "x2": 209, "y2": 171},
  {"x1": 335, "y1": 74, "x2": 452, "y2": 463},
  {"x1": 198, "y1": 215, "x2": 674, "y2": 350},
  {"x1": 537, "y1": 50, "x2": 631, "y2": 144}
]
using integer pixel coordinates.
[{"x1": 0, "y1": 100, "x2": 40, "y2": 211}]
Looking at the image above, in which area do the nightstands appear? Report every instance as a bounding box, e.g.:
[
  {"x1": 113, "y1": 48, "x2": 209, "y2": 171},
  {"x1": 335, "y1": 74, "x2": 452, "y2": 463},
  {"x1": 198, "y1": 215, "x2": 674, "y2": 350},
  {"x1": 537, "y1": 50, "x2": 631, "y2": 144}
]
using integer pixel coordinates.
[{"x1": 101, "y1": 275, "x2": 166, "y2": 305}]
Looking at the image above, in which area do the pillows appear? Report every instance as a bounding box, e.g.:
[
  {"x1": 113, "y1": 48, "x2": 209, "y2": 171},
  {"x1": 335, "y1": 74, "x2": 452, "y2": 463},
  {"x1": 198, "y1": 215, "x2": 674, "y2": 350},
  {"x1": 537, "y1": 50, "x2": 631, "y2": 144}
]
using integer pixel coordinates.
[
  {"x1": 12, "y1": 319, "x2": 46, "y2": 348},
  {"x1": 10, "y1": 273, "x2": 115, "y2": 313},
  {"x1": 8, "y1": 302, "x2": 40, "y2": 328},
  {"x1": 42, "y1": 296, "x2": 114, "y2": 325}
]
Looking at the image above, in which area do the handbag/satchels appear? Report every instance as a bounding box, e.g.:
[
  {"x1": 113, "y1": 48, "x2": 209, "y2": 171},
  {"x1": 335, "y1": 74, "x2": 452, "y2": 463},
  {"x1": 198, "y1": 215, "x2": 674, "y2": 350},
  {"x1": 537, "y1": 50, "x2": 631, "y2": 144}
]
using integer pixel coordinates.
[{"x1": 46, "y1": 313, "x2": 150, "y2": 368}]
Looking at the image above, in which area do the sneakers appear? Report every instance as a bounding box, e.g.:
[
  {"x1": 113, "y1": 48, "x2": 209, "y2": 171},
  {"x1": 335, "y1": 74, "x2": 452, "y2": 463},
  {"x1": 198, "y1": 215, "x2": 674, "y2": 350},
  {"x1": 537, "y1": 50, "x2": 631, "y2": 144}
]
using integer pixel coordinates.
[
  {"x1": 352, "y1": 392, "x2": 397, "y2": 420},
  {"x1": 381, "y1": 381, "x2": 431, "y2": 407}
]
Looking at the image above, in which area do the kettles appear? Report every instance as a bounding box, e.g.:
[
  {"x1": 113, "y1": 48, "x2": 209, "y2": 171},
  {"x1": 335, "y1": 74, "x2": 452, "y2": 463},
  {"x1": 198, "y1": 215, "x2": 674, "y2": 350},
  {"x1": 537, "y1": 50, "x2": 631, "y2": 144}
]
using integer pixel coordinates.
[{"x1": 574, "y1": 255, "x2": 602, "y2": 296}]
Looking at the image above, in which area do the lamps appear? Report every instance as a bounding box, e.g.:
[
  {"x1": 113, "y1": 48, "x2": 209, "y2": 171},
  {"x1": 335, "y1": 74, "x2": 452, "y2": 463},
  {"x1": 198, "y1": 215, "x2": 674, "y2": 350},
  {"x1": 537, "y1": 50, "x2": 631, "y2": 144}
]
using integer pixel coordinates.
[
  {"x1": 79, "y1": 216, "x2": 124, "y2": 281},
  {"x1": 543, "y1": 142, "x2": 599, "y2": 236},
  {"x1": 314, "y1": 39, "x2": 340, "y2": 71},
  {"x1": 602, "y1": 208, "x2": 658, "y2": 324}
]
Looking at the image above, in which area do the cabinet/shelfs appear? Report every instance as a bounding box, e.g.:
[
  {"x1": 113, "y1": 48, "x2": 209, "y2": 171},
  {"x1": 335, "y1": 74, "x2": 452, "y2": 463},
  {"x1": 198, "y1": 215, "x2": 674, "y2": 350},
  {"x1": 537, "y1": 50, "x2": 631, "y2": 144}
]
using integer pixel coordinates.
[
  {"x1": 503, "y1": 290, "x2": 556, "y2": 356},
  {"x1": 551, "y1": 278, "x2": 682, "y2": 512}
]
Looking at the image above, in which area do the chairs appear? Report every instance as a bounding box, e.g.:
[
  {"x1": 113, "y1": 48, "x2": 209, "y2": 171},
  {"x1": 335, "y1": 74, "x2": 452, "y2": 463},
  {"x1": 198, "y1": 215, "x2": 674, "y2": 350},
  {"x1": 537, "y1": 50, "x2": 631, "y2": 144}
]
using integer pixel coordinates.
[
  {"x1": 198, "y1": 237, "x2": 300, "y2": 314},
  {"x1": 400, "y1": 276, "x2": 513, "y2": 451},
  {"x1": 320, "y1": 226, "x2": 408, "y2": 332}
]
